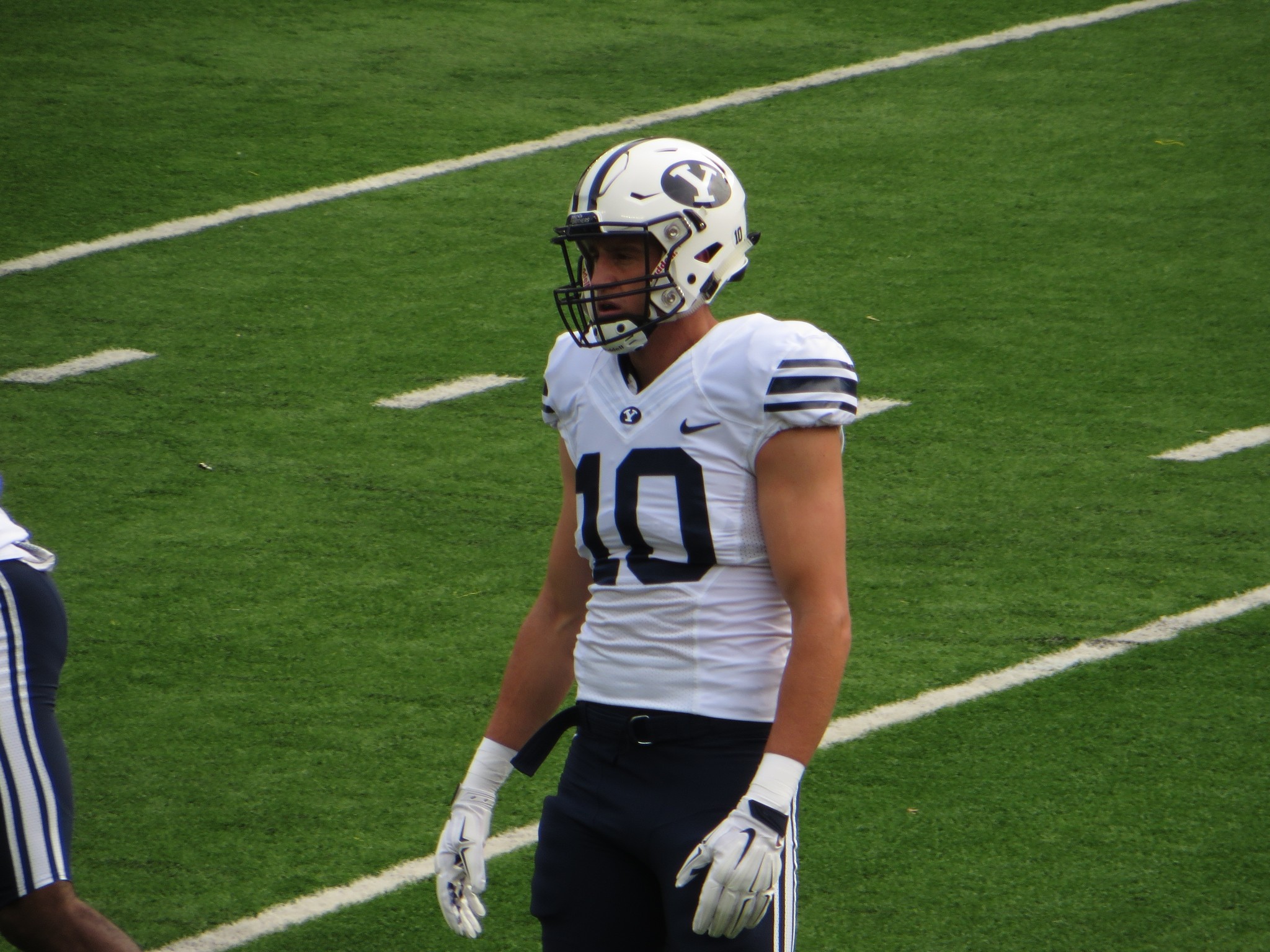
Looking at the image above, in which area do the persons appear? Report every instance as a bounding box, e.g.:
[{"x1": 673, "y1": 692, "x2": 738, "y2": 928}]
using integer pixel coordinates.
[
  {"x1": 428, "y1": 129, "x2": 862, "y2": 951},
  {"x1": 0, "y1": 499, "x2": 143, "y2": 952}
]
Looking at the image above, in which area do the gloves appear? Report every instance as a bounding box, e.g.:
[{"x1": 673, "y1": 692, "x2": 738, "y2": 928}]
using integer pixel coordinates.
[
  {"x1": 434, "y1": 784, "x2": 497, "y2": 939},
  {"x1": 675, "y1": 795, "x2": 789, "y2": 940}
]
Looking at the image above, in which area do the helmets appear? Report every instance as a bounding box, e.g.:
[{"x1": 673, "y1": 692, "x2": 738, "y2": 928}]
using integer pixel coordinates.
[{"x1": 564, "y1": 136, "x2": 748, "y2": 314}]
